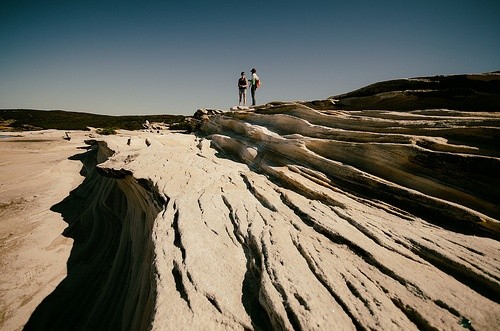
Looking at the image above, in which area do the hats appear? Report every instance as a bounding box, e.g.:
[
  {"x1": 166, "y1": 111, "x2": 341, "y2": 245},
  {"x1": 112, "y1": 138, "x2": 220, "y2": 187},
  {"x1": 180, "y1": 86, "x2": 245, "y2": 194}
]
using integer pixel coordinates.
[
  {"x1": 241, "y1": 72, "x2": 245, "y2": 75},
  {"x1": 250, "y1": 68, "x2": 256, "y2": 72}
]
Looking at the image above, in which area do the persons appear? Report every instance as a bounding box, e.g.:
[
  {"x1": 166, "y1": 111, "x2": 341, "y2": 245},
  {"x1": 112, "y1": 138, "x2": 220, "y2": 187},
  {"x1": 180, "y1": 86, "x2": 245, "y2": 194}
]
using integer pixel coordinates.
[
  {"x1": 247, "y1": 67, "x2": 260, "y2": 106},
  {"x1": 238, "y1": 71, "x2": 248, "y2": 106}
]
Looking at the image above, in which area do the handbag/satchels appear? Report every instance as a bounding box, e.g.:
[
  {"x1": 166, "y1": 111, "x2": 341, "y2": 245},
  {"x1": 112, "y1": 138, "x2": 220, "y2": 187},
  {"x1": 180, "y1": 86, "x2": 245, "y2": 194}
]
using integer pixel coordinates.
[{"x1": 255, "y1": 79, "x2": 260, "y2": 88}]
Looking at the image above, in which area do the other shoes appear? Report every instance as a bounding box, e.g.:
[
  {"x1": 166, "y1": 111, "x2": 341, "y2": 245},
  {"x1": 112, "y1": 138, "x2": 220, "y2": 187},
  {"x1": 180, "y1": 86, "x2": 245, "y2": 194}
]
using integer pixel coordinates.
[{"x1": 251, "y1": 105, "x2": 255, "y2": 107}]
